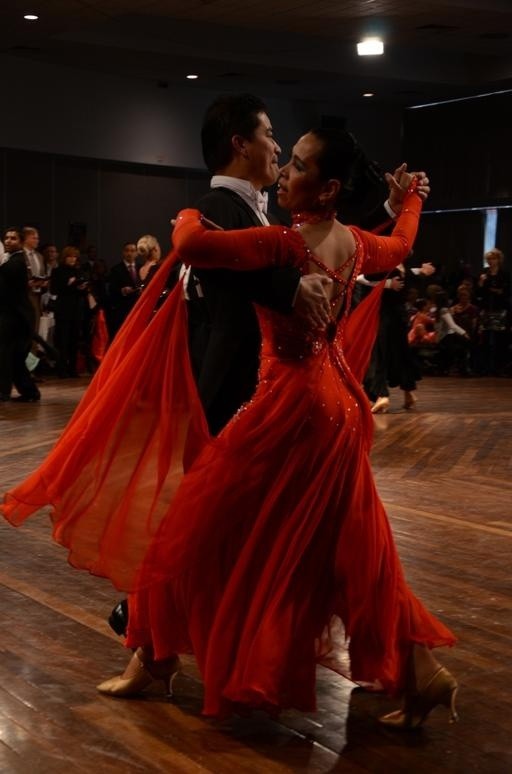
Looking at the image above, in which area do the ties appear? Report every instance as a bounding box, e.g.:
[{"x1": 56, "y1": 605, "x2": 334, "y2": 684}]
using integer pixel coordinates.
[
  {"x1": 128, "y1": 263, "x2": 136, "y2": 281},
  {"x1": 29, "y1": 253, "x2": 37, "y2": 275}
]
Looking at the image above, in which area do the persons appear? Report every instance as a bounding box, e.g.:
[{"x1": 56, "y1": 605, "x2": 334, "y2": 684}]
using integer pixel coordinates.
[
  {"x1": 95, "y1": 121, "x2": 460, "y2": 730},
  {"x1": 2, "y1": 223, "x2": 512, "y2": 412},
  {"x1": 106, "y1": 95, "x2": 435, "y2": 638}
]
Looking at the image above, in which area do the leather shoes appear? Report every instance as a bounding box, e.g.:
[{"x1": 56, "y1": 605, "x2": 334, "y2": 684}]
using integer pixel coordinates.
[
  {"x1": 15, "y1": 392, "x2": 40, "y2": 403},
  {"x1": 109, "y1": 601, "x2": 180, "y2": 678}
]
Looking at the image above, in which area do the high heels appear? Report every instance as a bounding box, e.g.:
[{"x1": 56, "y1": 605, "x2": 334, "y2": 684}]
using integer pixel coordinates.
[
  {"x1": 379, "y1": 661, "x2": 458, "y2": 729},
  {"x1": 96, "y1": 656, "x2": 177, "y2": 696},
  {"x1": 371, "y1": 397, "x2": 389, "y2": 413},
  {"x1": 402, "y1": 393, "x2": 417, "y2": 409}
]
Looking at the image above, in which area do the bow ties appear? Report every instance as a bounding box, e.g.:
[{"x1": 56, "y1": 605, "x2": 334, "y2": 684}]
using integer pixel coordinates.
[{"x1": 256, "y1": 191, "x2": 268, "y2": 214}]
